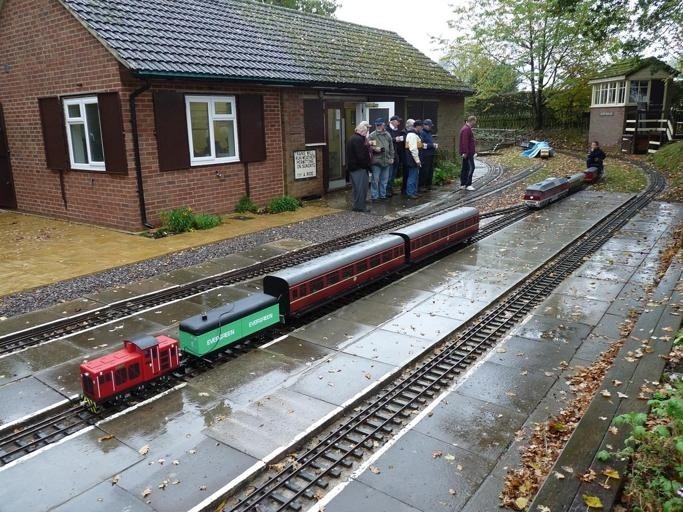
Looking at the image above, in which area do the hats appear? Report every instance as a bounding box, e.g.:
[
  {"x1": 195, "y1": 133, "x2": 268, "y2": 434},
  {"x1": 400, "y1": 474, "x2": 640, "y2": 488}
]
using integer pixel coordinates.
[{"x1": 360, "y1": 115, "x2": 434, "y2": 127}]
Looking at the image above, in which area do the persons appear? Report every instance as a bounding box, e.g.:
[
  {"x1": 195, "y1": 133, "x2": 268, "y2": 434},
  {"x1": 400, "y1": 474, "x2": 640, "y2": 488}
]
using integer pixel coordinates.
[
  {"x1": 344, "y1": 114, "x2": 440, "y2": 213},
  {"x1": 457, "y1": 115, "x2": 477, "y2": 192},
  {"x1": 585, "y1": 140, "x2": 607, "y2": 181}
]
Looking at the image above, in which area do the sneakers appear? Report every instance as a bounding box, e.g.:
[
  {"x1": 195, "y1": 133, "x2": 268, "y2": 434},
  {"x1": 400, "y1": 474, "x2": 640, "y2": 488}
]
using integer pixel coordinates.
[
  {"x1": 459, "y1": 184, "x2": 476, "y2": 190},
  {"x1": 369, "y1": 198, "x2": 390, "y2": 202},
  {"x1": 352, "y1": 207, "x2": 371, "y2": 212},
  {"x1": 401, "y1": 187, "x2": 432, "y2": 199}
]
